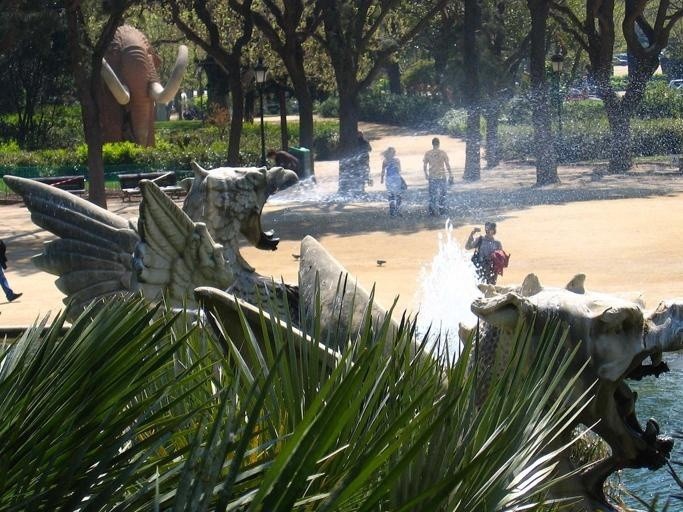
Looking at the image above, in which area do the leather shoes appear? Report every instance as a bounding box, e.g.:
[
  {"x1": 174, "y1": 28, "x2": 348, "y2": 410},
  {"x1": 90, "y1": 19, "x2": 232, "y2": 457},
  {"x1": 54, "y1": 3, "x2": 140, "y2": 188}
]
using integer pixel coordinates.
[{"x1": 7, "y1": 292, "x2": 23, "y2": 302}]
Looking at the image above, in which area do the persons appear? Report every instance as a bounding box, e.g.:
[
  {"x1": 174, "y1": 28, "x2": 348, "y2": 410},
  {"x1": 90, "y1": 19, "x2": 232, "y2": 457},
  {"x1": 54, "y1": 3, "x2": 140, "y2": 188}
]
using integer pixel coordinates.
[
  {"x1": 357, "y1": 132, "x2": 373, "y2": 192},
  {"x1": 267, "y1": 150, "x2": 301, "y2": 177},
  {"x1": 423, "y1": 138, "x2": 453, "y2": 215},
  {"x1": 465, "y1": 222, "x2": 502, "y2": 285},
  {"x1": 0, "y1": 240, "x2": 22, "y2": 301},
  {"x1": 380, "y1": 148, "x2": 403, "y2": 217}
]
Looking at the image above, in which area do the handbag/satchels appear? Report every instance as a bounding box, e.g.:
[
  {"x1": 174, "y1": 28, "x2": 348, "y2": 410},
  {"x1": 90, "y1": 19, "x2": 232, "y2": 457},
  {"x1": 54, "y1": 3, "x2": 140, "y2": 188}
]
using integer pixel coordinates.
[{"x1": 471, "y1": 252, "x2": 482, "y2": 269}]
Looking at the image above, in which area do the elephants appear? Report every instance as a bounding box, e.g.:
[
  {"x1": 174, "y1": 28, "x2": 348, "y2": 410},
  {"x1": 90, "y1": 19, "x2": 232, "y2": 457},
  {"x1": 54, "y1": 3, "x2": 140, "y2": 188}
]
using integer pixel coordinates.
[{"x1": 65, "y1": 24, "x2": 189, "y2": 150}]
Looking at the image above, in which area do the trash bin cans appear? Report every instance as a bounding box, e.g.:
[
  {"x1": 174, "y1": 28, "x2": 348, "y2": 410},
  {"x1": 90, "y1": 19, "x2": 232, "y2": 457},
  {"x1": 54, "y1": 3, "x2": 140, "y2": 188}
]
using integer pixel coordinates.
[{"x1": 287, "y1": 147, "x2": 311, "y2": 182}]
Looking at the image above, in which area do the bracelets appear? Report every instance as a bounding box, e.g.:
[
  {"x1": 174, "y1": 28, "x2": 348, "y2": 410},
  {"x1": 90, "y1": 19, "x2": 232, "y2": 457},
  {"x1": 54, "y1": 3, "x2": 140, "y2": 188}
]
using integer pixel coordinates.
[{"x1": 469, "y1": 237, "x2": 474, "y2": 241}]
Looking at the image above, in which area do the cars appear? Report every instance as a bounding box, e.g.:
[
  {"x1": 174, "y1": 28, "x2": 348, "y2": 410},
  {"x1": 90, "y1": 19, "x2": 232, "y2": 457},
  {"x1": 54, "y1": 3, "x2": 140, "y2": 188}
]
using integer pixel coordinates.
[
  {"x1": 612, "y1": 57, "x2": 628, "y2": 66},
  {"x1": 669, "y1": 80, "x2": 683, "y2": 89}
]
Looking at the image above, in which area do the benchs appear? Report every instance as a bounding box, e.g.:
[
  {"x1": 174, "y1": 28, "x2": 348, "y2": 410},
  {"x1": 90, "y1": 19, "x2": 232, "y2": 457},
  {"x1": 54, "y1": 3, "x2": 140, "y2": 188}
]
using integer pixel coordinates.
[{"x1": 26, "y1": 169, "x2": 188, "y2": 205}]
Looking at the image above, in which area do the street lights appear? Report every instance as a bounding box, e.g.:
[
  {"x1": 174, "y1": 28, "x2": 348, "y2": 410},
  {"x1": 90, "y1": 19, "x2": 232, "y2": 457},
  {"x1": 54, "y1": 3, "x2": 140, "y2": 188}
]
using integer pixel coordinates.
[
  {"x1": 196, "y1": 61, "x2": 204, "y2": 117},
  {"x1": 551, "y1": 46, "x2": 567, "y2": 133},
  {"x1": 252, "y1": 56, "x2": 269, "y2": 164}
]
